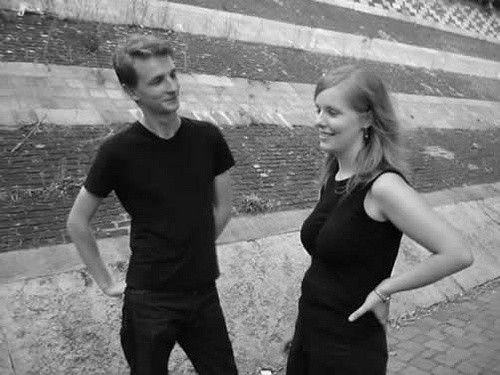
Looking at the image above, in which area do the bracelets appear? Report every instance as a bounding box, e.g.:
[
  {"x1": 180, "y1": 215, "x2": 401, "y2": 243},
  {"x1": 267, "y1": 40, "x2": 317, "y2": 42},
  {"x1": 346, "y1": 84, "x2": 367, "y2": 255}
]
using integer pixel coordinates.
[{"x1": 373, "y1": 287, "x2": 390, "y2": 303}]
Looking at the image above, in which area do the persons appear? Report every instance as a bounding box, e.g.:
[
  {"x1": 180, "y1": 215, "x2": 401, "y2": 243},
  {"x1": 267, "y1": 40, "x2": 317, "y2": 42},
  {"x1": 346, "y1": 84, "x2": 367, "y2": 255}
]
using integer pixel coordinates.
[
  {"x1": 286, "y1": 65, "x2": 474, "y2": 375},
  {"x1": 67, "y1": 35, "x2": 240, "y2": 375}
]
